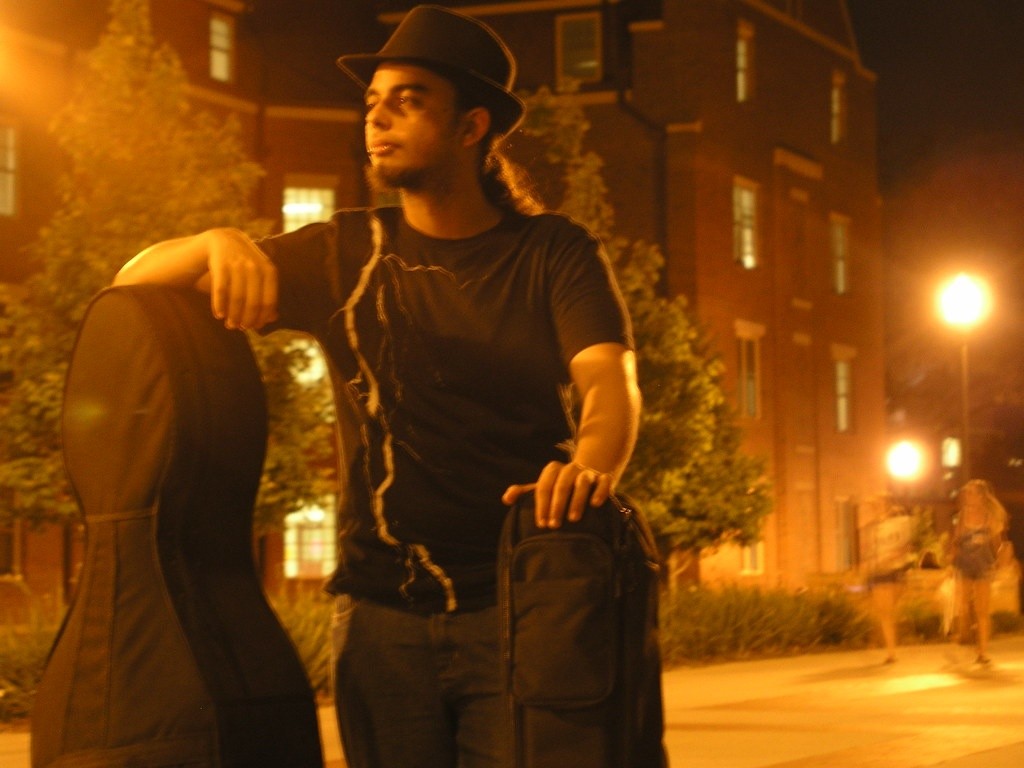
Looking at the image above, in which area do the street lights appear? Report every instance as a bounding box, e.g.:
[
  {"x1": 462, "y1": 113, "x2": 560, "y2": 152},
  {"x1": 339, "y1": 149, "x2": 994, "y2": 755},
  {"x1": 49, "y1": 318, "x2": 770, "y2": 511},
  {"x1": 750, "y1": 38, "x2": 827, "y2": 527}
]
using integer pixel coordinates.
[{"x1": 936, "y1": 271, "x2": 992, "y2": 484}]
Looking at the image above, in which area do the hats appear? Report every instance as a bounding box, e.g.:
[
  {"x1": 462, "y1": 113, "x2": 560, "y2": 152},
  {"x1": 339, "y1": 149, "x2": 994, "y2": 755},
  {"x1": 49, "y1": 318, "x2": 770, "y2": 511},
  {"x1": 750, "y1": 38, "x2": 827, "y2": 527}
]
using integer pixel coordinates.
[{"x1": 337, "y1": 5, "x2": 526, "y2": 143}]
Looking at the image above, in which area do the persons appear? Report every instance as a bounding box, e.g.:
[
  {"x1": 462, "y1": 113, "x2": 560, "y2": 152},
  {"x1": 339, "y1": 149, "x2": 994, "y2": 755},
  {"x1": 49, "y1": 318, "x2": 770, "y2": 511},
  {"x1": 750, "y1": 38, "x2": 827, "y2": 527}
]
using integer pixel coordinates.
[
  {"x1": 109, "y1": 2, "x2": 644, "y2": 768},
  {"x1": 869, "y1": 474, "x2": 1023, "y2": 671}
]
